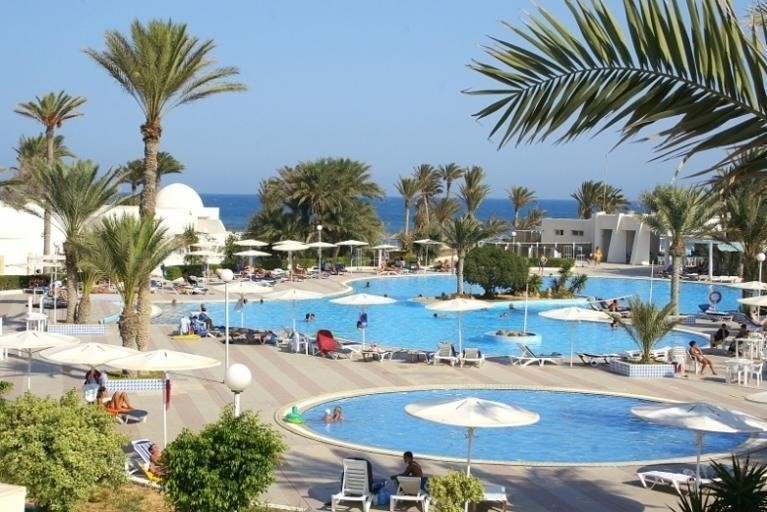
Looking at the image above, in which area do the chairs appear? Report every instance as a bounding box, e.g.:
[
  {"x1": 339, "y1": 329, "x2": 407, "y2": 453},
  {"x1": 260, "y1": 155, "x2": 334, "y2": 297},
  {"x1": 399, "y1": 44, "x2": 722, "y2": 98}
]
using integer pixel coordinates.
[
  {"x1": 684, "y1": 350, "x2": 700, "y2": 376},
  {"x1": 737, "y1": 363, "x2": 764, "y2": 388},
  {"x1": 634, "y1": 462, "x2": 736, "y2": 498},
  {"x1": 316, "y1": 330, "x2": 352, "y2": 362},
  {"x1": 128, "y1": 438, "x2": 162, "y2": 483},
  {"x1": 509, "y1": 342, "x2": 620, "y2": 368},
  {"x1": 434, "y1": 342, "x2": 485, "y2": 369},
  {"x1": 330, "y1": 457, "x2": 432, "y2": 512}
]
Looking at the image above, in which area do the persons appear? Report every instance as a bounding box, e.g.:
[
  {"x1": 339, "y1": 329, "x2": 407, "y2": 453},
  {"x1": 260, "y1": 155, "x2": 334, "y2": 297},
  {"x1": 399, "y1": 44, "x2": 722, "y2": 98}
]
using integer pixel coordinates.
[
  {"x1": 507, "y1": 303, "x2": 515, "y2": 310},
  {"x1": 418, "y1": 293, "x2": 422, "y2": 298},
  {"x1": 712, "y1": 323, "x2": 729, "y2": 347},
  {"x1": 389, "y1": 451, "x2": 423, "y2": 493},
  {"x1": 322, "y1": 408, "x2": 332, "y2": 423},
  {"x1": 332, "y1": 406, "x2": 343, "y2": 423},
  {"x1": 148, "y1": 442, "x2": 167, "y2": 466},
  {"x1": 609, "y1": 298, "x2": 622, "y2": 327},
  {"x1": 259, "y1": 298, "x2": 264, "y2": 304},
  {"x1": 198, "y1": 303, "x2": 214, "y2": 330},
  {"x1": 441, "y1": 291, "x2": 446, "y2": 297},
  {"x1": 190, "y1": 312, "x2": 214, "y2": 338},
  {"x1": 500, "y1": 312, "x2": 509, "y2": 320},
  {"x1": 383, "y1": 293, "x2": 387, "y2": 297},
  {"x1": 433, "y1": 311, "x2": 441, "y2": 317},
  {"x1": 310, "y1": 314, "x2": 316, "y2": 321},
  {"x1": 748, "y1": 310, "x2": 766, "y2": 325},
  {"x1": 364, "y1": 281, "x2": 370, "y2": 288},
  {"x1": 730, "y1": 323, "x2": 749, "y2": 357},
  {"x1": 628, "y1": 400, "x2": 766, "y2": 497},
  {"x1": 688, "y1": 338, "x2": 717, "y2": 377},
  {"x1": 96, "y1": 385, "x2": 133, "y2": 414},
  {"x1": 593, "y1": 246, "x2": 603, "y2": 266},
  {"x1": 305, "y1": 313, "x2": 311, "y2": 321}
]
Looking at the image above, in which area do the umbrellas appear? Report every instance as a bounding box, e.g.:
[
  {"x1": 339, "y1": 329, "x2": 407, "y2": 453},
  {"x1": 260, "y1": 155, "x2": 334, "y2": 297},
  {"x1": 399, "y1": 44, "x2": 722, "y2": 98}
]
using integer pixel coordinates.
[
  {"x1": 403, "y1": 393, "x2": 540, "y2": 512},
  {"x1": 727, "y1": 280, "x2": 767, "y2": 294},
  {"x1": 212, "y1": 280, "x2": 272, "y2": 324},
  {"x1": 188, "y1": 235, "x2": 443, "y2": 284},
  {"x1": 536, "y1": 302, "x2": 616, "y2": 368},
  {"x1": 106, "y1": 348, "x2": 223, "y2": 448},
  {"x1": 426, "y1": 294, "x2": 491, "y2": 369},
  {"x1": 736, "y1": 295, "x2": 766, "y2": 311},
  {"x1": 262, "y1": 284, "x2": 321, "y2": 330},
  {"x1": 1, "y1": 327, "x2": 81, "y2": 394},
  {"x1": 39, "y1": 339, "x2": 140, "y2": 385},
  {"x1": 330, "y1": 289, "x2": 397, "y2": 345}
]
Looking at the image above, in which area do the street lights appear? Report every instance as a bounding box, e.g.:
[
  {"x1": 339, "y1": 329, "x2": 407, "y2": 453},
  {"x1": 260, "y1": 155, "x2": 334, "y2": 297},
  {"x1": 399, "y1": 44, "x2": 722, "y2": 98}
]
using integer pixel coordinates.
[
  {"x1": 510, "y1": 231, "x2": 517, "y2": 253},
  {"x1": 316, "y1": 224, "x2": 324, "y2": 281},
  {"x1": 754, "y1": 252, "x2": 767, "y2": 333},
  {"x1": 221, "y1": 362, "x2": 251, "y2": 420},
  {"x1": 218, "y1": 267, "x2": 235, "y2": 386}
]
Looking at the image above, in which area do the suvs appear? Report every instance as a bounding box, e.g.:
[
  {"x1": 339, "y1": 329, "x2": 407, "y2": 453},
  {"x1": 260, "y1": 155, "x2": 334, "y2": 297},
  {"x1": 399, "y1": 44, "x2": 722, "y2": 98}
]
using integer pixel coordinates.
[{"x1": 23, "y1": 286, "x2": 68, "y2": 308}]
